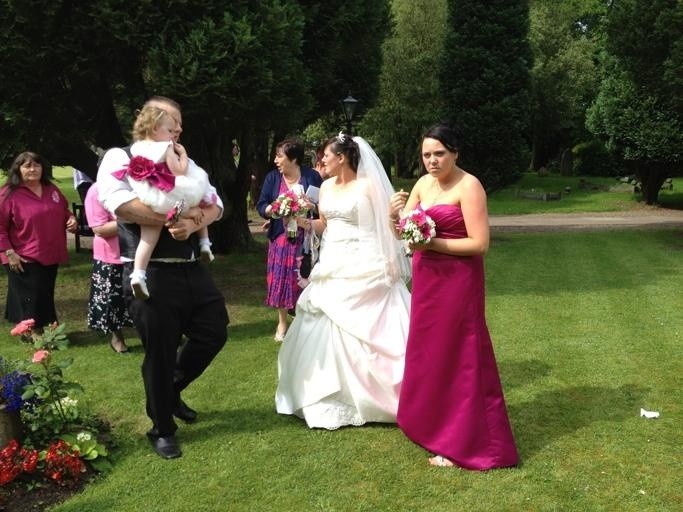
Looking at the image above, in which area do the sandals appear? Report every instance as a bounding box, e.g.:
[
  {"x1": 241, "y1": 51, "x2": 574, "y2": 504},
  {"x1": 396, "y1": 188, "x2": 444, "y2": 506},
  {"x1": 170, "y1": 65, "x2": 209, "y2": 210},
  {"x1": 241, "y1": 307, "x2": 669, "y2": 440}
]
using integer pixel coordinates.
[
  {"x1": 275, "y1": 326, "x2": 286, "y2": 340},
  {"x1": 429, "y1": 455, "x2": 453, "y2": 466}
]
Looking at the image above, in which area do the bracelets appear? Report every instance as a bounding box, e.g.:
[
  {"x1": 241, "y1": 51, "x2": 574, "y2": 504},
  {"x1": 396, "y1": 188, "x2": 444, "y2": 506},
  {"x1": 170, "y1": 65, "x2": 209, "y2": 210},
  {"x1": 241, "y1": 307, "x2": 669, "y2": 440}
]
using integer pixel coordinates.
[
  {"x1": 388, "y1": 216, "x2": 399, "y2": 222},
  {"x1": 5, "y1": 249, "x2": 14, "y2": 256}
]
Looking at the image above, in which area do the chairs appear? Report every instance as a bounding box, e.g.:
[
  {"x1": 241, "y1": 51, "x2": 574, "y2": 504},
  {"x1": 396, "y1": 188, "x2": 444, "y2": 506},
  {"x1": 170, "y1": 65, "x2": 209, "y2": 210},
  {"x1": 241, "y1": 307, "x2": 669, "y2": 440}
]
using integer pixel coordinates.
[{"x1": 72, "y1": 202, "x2": 95, "y2": 253}]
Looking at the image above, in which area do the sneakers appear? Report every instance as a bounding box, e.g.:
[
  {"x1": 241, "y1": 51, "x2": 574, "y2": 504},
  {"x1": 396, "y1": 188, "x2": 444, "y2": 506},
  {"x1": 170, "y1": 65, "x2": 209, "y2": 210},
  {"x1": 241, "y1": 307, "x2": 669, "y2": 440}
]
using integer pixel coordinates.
[
  {"x1": 201, "y1": 245, "x2": 214, "y2": 263},
  {"x1": 131, "y1": 278, "x2": 150, "y2": 300}
]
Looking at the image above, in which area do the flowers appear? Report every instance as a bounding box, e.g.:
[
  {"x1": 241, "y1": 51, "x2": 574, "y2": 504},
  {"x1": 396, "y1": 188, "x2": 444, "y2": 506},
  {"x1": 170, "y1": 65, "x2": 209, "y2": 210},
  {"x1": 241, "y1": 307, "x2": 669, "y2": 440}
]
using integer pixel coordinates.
[
  {"x1": 166, "y1": 197, "x2": 185, "y2": 223},
  {"x1": 399, "y1": 208, "x2": 436, "y2": 258},
  {"x1": 271, "y1": 189, "x2": 316, "y2": 238}
]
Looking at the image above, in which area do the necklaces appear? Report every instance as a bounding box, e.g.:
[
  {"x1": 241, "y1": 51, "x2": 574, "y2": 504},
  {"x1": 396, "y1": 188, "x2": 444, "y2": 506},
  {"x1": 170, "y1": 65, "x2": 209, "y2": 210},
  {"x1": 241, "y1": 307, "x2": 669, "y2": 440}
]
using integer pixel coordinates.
[{"x1": 433, "y1": 182, "x2": 447, "y2": 203}]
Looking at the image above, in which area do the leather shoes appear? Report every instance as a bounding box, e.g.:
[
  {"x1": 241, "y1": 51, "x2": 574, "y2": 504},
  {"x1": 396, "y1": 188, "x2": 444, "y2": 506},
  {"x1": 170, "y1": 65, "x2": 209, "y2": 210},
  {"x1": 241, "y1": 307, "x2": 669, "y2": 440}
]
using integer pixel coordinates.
[
  {"x1": 153, "y1": 436, "x2": 182, "y2": 458},
  {"x1": 174, "y1": 399, "x2": 197, "y2": 422},
  {"x1": 111, "y1": 344, "x2": 129, "y2": 353}
]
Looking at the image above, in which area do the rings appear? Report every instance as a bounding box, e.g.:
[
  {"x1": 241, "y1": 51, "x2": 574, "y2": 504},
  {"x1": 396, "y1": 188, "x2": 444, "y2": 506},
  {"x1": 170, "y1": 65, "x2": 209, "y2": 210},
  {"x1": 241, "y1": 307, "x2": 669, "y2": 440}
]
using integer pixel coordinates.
[{"x1": 12, "y1": 266, "x2": 15, "y2": 271}]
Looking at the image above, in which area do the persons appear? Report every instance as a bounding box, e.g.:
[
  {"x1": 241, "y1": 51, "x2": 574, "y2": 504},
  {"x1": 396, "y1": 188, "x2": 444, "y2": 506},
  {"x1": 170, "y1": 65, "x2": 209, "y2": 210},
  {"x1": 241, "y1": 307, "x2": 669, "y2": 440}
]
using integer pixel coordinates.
[
  {"x1": 312, "y1": 144, "x2": 331, "y2": 180},
  {"x1": 84, "y1": 149, "x2": 136, "y2": 352},
  {"x1": 109, "y1": 102, "x2": 215, "y2": 301},
  {"x1": 256, "y1": 138, "x2": 324, "y2": 339},
  {"x1": 71, "y1": 165, "x2": 92, "y2": 206},
  {"x1": 387, "y1": 125, "x2": 519, "y2": 469},
  {"x1": 95, "y1": 95, "x2": 230, "y2": 461},
  {"x1": 275, "y1": 131, "x2": 412, "y2": 431},
  {"x1": 231, "y1": 143, "x2": 256, "y2": 223},
  {"x1": 0, "y1": 152, "x2": 77, "y2": 337}
]
